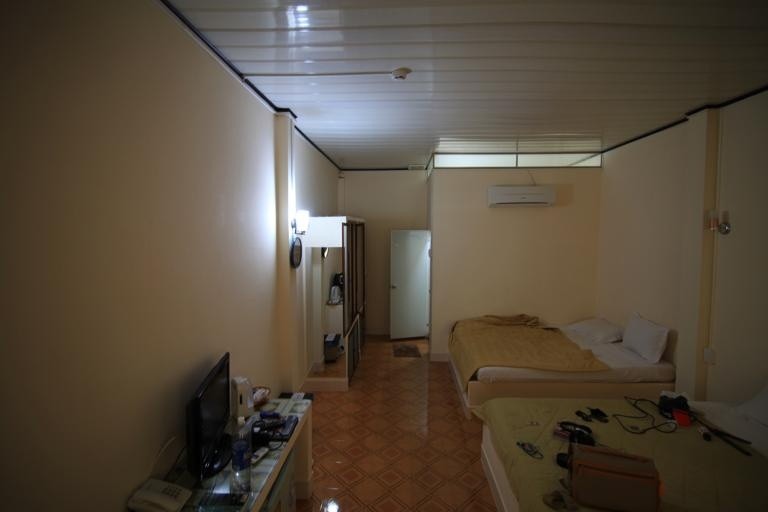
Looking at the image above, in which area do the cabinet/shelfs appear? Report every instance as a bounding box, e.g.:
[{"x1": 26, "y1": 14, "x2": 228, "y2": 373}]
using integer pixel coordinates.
[
  {"x1": 306, "y1": 217, "x2": 368, "y2": 389},
  {"x1": 229, "y1": 416, "x2": 252, "y2": 492}
]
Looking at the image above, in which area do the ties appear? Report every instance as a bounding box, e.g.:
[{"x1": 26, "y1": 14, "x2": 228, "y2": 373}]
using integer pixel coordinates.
[{"x1": 184, "y1": 352, "x2": 234, "y2": 478}]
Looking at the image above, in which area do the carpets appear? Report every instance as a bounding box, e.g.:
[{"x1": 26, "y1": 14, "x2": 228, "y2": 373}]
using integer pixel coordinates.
[{"x1": 393, "y1": 341, "x2": 421, "y2": 357}]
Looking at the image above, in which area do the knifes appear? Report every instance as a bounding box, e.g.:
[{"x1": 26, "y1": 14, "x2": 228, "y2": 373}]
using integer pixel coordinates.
[{"x1": 487, "y1": 183, "x2": 555, "y2": 207}]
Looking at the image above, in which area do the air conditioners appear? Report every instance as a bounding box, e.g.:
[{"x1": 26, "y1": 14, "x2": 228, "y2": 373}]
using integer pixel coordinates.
[{"x1": 487, "y1": 183, "x2": 555, "y2": 207}]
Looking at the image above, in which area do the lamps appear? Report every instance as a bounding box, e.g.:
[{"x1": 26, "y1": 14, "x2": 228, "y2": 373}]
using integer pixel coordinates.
[
  {"x1": 292, "y1": 209, "x2": 311, "y2": 235},
  {"x1": 708, "y1": 210, "x2": 732, "y2": 236}
]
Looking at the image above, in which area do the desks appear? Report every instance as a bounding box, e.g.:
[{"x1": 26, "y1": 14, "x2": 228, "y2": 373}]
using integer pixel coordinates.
[{"x1": 165, "y1": 395, "x2": 314, "y2": 512}]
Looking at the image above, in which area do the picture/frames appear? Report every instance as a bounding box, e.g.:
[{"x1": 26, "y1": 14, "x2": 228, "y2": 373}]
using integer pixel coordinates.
[{"x1": 165, "y1": 395, "x2": 314, "y2": 512}]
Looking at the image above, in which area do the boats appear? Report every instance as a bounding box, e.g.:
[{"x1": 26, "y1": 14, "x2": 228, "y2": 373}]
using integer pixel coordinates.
[{"x1": 231, "y1": 376, "x2": 254, "y2": 420}]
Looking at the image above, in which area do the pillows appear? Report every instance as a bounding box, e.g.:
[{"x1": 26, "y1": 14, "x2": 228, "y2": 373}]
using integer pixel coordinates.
[{"x1": 568, "y1": 309, "x2": 670, "y2": 362}]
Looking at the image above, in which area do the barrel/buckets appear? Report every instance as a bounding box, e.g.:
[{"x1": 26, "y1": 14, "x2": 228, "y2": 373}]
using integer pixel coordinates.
[
  {"x1": 522, "y1": 443, "x2": 543, "y2": 461},
  {"x1": 543, "y1": 421, "x2": 591, "y2": 511}
]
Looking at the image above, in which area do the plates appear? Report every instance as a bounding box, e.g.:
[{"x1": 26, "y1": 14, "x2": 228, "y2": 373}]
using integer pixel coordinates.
[{"x1": 306, "y1": 217, "x2": 368, "y2": 389}]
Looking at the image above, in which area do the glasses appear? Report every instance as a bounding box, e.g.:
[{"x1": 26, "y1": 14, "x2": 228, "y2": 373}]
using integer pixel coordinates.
[
  {"x1": 708, "y1": 210, "x2": 732, "y2": 236},
  {"x1": 292, "y1": 209, "x2": 311, "y2": 235}
]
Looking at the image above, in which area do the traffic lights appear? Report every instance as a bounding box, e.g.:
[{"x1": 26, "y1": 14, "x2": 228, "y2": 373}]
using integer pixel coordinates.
[{"x1": 568, "y1": 309, "x2": 670, "y2": 362}]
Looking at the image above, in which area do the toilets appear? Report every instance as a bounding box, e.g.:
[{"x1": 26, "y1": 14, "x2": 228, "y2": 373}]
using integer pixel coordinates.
[{"x1": 128, "y1": 478, "x2": 192, "y2": 512}]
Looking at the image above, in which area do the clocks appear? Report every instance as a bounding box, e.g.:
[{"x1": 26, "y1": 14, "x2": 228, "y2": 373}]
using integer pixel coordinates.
[{"x1": 291, "y1": 236, "x2": 302, "y2": 268}]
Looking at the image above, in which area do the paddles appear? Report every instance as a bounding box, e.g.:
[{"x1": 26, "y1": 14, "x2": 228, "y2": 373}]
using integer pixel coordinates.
[{"x1": 393, "y1": 341, "x2": 421, "y2": 357}]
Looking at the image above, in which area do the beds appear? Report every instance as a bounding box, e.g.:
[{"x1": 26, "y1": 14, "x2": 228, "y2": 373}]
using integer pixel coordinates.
[{"x1": 449, "y1": 322, "x2": 768, "y2": 510}]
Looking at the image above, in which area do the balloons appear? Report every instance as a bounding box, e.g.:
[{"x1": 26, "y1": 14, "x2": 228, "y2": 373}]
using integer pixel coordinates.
[{"x1": 291, "y1": 236, "x2": 302, "y2": 268}]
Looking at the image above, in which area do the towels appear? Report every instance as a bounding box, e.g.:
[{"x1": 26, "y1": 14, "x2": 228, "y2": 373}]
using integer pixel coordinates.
[{"x1": 324, "y1": 333, "x2": 341, "y2": 363}]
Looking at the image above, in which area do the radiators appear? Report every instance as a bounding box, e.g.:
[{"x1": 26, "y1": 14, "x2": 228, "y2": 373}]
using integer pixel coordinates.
[{"x1": 251, "y1": 447, "x2": 270, "y2": 464}]
